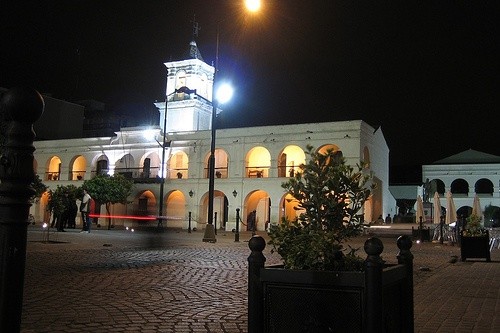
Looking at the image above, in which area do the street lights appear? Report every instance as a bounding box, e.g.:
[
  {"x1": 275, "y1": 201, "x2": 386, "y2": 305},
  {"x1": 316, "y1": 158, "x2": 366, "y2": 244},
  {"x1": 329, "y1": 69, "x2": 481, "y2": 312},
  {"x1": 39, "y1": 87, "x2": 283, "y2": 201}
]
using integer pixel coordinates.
[{"x1": 202, "y1": 0, "x2": 262, "y2": 242}]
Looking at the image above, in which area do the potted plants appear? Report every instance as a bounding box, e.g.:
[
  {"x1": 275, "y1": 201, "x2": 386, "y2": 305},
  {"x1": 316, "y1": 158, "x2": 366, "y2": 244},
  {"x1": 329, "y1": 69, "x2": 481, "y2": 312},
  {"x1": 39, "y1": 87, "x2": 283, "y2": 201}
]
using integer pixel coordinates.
[
  {"x1": 460, "y1": 210, "x2": 491, "y2": 261},
  {"x1": 247, "y1": 144, "x2": 414, "y2": 333}
]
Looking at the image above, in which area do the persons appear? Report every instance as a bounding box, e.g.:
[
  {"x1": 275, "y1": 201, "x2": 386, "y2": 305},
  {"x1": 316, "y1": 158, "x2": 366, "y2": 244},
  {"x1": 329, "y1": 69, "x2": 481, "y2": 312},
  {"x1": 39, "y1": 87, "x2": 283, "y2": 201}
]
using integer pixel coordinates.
[
  {"x1": 247, "y1": 210, "x2": 257, "y2": 231},
  {"x1": 377, "y1": 211, "x2": 412, "y2": 224},
  {"x1": 51, "y1": 189, "x2": 92, "y2": 234}
]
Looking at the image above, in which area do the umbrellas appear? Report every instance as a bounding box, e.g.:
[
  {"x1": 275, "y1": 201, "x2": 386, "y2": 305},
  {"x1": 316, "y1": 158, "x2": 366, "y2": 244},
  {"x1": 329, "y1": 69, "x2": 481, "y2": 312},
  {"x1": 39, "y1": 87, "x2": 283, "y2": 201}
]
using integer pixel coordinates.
[{"x1": 416, "y1": 191, "x2": 484, "y2": 243}]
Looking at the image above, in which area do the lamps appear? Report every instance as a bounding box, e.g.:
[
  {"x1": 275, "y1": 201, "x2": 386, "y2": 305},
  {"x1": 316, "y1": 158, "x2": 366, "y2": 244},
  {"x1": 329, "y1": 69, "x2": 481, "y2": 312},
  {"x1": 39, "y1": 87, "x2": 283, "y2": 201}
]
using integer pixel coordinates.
[
  {"x1": 189, "y1": 190, "x2": 193, "y2": 197},
  {"x1": 233, "y1": 189, "x2": 237, "y2": 198}
]
